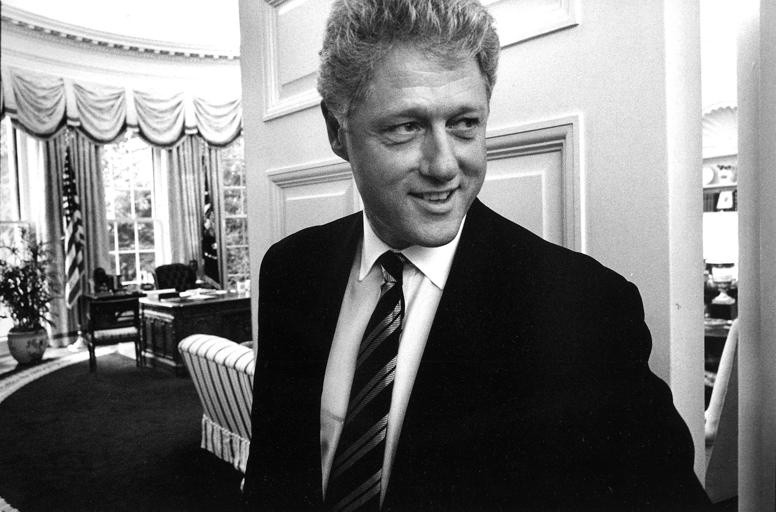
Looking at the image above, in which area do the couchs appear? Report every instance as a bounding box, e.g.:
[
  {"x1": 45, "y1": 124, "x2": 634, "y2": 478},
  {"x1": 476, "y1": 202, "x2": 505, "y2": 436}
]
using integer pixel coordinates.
[{"x1": 176, "y1": 332, "x2": 254, "y2": 475}]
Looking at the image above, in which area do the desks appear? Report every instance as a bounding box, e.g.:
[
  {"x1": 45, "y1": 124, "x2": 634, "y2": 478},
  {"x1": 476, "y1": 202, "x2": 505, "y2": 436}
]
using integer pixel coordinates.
[{"x1": 137, "y1": 289, "x2": 252, "y2": 374}]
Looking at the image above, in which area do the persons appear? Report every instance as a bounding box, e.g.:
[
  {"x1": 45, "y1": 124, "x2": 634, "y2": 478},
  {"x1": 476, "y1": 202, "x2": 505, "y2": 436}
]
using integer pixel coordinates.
[{"x1": 243, "y1": 1, "x2": 716, "y2": 511}]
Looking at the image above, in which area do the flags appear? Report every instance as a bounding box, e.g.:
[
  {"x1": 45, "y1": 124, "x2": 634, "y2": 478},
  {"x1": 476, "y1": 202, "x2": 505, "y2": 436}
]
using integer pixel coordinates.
[
  {"x1": 199, "y1": 157, "x2": 223, "y2": 290},
  {"x1": 59, "y1": 145, "x2": 85, "y2": 311}
]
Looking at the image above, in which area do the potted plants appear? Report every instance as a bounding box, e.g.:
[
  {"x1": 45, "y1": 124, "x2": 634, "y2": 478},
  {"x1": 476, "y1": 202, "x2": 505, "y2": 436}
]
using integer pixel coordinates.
[{"x1": 0, "y1": 225, "x2": 66, "y2": 364}]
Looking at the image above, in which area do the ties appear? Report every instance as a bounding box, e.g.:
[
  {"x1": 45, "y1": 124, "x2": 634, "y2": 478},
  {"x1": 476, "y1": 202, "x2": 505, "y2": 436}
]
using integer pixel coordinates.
[{"x1": 322, "y1": 252, "x2": 405, "y2": 511}]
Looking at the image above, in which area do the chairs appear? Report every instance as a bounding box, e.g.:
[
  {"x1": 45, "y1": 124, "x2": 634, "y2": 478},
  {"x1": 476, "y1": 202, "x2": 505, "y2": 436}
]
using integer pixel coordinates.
[
  {"x1": 151, "y1": 259, "x2": 198, "y2": 301},
  {"x1": 75, "y1": 288, "x2": 146, "y2": 374}
]
